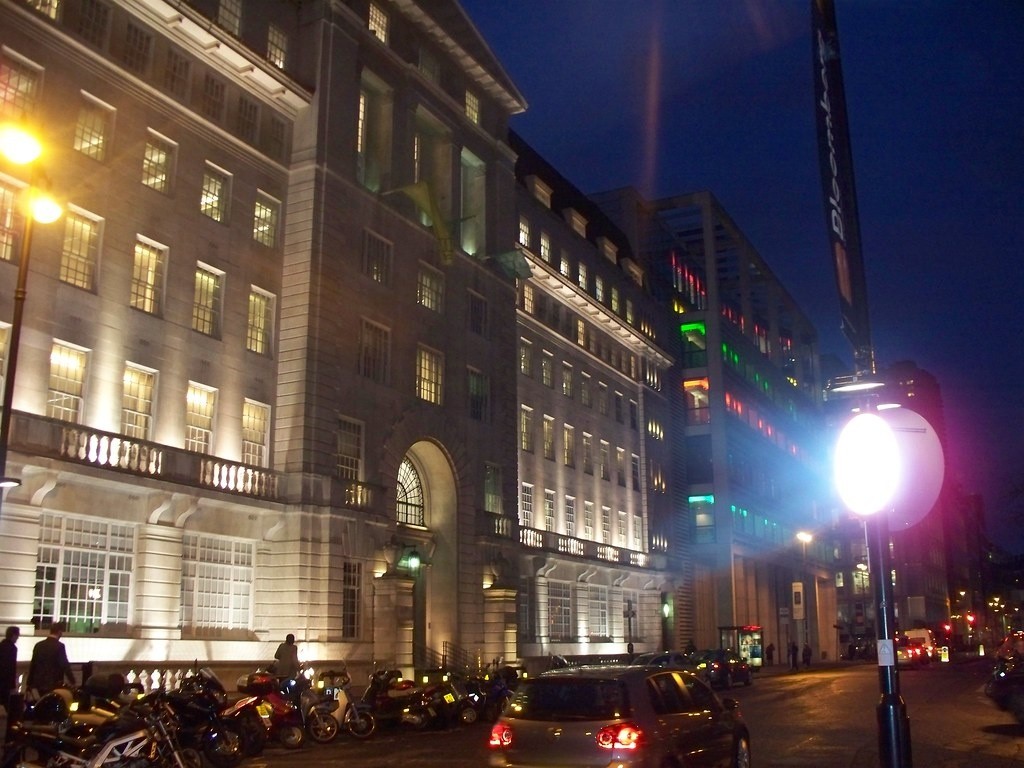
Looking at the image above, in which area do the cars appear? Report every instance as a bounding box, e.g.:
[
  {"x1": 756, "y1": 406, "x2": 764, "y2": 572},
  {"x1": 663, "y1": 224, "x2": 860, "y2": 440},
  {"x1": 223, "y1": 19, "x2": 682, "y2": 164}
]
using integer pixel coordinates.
[
  {"x1": 488, "y1": 664, "x2": 751, "y2": 768},
  {"x1": 689, "y1": 650, "x2": 752, "y2": 689},
  {"x1": 630, "y1": 652, "x2": 686, "y2": 664},
  {"x1": 893, "y1": 634, "x2": 930, "y2": 668}
]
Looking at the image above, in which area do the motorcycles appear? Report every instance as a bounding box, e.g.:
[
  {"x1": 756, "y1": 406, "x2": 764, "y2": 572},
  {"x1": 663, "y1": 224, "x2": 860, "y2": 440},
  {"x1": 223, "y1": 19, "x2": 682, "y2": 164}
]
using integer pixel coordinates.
[
  {"x1": 14, "y1": 657, "x2": 520, "y2": 768},
  {"x1": 985, "y1": 653, "x2": 1014, "y2": 698}
]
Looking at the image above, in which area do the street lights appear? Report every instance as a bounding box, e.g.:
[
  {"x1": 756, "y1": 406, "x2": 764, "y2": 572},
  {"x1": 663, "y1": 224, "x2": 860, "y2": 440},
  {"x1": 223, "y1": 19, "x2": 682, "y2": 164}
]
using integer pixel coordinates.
[
  {"x1": 833, "y1": 412, "x2": 914, "y2": 768},
  {"x1": 797, "y1": 532, "x2": 814, "y2": 668}
]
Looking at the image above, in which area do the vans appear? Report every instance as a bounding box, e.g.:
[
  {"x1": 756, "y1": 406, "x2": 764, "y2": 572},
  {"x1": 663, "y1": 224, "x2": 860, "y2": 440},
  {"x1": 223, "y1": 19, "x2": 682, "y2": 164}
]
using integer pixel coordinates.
[{"x1": 904, "y1": 629, "x2": 940, "y2": 661}]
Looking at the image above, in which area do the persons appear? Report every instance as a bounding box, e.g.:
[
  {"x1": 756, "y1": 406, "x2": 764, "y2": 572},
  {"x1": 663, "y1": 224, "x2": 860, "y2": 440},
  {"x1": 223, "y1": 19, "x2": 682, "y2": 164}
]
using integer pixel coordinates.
[
  {"x1": 766, "y1": 644, "x2": 775, "y2": 667},
  {"x1": 0, "y1": 626, "x2": 20, "y2": 706},
  {"x1": 791, "y1": 642, "x2": 799, "y2": 672},
  {"x1": 27, "y1": 622, "x2": 75, "y2": 698},
  {"x1": 685, "y1": 640, "x2": 696, "y2": 654},
  {"x1": 802, "y1": 641, "x2": 812, "y2": 664},
  {"x1": 274, "y1": 634, "x2": 300, "y2": 683}
]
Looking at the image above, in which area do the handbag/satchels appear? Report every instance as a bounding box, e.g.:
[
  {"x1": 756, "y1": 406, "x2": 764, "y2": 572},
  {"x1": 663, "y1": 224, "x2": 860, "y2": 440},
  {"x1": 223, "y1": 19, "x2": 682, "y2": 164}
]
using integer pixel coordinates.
[{"x1": 23, "y1": 687, "x2": 41, "y2": 704}]
